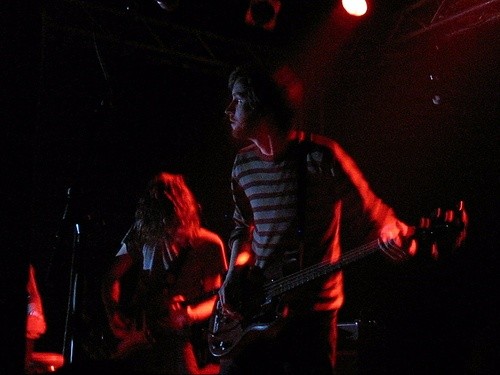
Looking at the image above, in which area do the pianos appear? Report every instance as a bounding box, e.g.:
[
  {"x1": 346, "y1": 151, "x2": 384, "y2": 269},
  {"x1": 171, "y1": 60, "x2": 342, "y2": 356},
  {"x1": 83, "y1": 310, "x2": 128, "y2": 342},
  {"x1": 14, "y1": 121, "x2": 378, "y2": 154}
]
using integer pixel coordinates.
[{"x1": 241, "y1": 318, "x2": 387, "y2": 351}]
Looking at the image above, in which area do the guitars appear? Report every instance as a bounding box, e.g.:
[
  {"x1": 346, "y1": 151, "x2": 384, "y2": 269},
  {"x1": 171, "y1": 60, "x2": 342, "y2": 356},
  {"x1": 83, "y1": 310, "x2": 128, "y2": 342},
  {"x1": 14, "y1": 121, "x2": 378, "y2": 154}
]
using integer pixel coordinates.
[
  {"x1": 206, "y1": 201, "x2": 468, "y2": 357},
  {"x1": 71, "y1": 286, "x2": 220, "y2": 362}
]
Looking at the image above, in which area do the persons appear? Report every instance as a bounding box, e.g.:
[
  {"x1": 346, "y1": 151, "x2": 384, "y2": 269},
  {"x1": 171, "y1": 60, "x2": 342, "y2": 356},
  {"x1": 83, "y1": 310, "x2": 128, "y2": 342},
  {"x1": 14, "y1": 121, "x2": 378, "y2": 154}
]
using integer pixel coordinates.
[
  {"x1": 101, "y1": 173, "x2": 229, "y2": 375},
  {"x1": 218, "y1": 58, "x2": 417, "y2": 375}
]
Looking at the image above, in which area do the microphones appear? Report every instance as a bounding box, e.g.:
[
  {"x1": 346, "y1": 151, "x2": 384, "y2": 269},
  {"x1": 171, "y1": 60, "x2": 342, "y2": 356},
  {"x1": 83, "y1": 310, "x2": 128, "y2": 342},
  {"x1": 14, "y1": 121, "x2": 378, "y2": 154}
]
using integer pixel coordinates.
[{"x1": 56, "y1": 187, "x2": 74, "y2": 238}]
